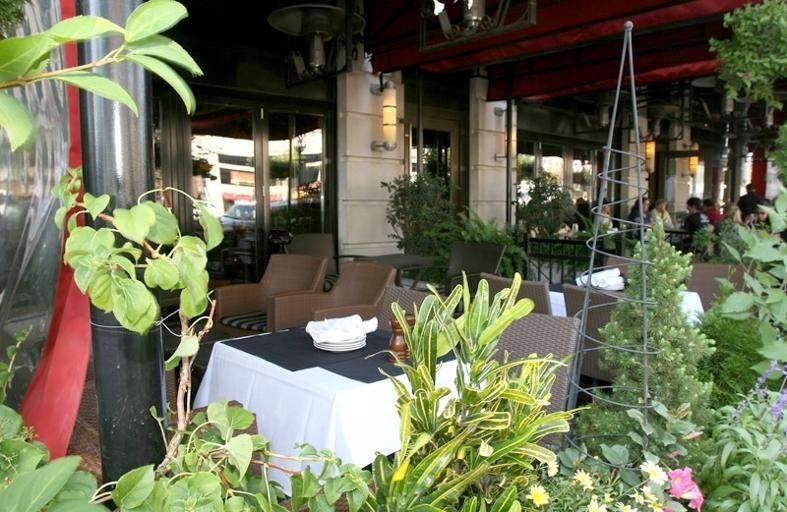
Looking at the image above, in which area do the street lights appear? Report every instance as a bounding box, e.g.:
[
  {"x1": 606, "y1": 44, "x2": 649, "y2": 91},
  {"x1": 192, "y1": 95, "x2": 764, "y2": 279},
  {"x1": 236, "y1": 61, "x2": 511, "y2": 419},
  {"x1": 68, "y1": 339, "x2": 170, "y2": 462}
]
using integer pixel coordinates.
[{"x1": 293, "y1": 140, "x2": 306, "y2": 203}]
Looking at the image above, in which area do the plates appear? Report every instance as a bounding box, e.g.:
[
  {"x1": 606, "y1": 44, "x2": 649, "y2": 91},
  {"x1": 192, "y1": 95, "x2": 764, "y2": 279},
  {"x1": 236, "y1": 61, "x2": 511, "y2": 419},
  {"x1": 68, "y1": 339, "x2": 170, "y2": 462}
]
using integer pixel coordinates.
[{"x1": 313, "y1": 333, "x2": 367, "y2": 352}]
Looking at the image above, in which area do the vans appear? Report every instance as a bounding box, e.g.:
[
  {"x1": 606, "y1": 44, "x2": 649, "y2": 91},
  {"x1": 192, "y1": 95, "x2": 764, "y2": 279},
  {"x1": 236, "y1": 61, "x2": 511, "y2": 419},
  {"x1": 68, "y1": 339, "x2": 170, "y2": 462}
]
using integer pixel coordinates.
[{"x1": 220, "y1": 202, "x2": 286, "y2": 229}]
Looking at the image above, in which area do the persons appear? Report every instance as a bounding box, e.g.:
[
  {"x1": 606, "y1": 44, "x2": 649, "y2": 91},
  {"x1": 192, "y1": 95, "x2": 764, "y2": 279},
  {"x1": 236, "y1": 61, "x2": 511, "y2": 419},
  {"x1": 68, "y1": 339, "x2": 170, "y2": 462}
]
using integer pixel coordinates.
[
  {"x1": 680, "y1": 184, "x2": 782, "y2": 264},
  {"x1": 574, "y1": 196, "x2": 613, "y2": 239},
  {"x1": 650, "y1": 199, "x2": 674, "y2": 242},
  {"x1": 626, "y1": 197, "x2": 653, "y2": 240}
]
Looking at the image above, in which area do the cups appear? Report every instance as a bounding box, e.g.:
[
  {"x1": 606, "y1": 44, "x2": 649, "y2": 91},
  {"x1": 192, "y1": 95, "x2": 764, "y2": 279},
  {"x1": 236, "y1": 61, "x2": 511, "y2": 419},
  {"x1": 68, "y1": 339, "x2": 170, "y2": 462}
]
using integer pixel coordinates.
[{"x1": 572, "y1": 223, "x2": 579, "y2": 233}]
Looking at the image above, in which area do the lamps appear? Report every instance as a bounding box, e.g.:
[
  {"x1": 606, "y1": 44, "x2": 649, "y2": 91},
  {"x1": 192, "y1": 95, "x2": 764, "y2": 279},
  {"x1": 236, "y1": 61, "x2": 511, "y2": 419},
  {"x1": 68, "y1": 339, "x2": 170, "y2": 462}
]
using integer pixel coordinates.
[
  {"x1": 370, "y1": 81, "x2": 398, "y2": 151},
  {"x1": 639, "y1": 136, "x2": 656, "y2": 172},
  {"x1": 495, "y1": 104, "x2": 517, "y2": 161},
  {"x1": 681, "y1": 143, "x2": 699, "y2": 176}
]
